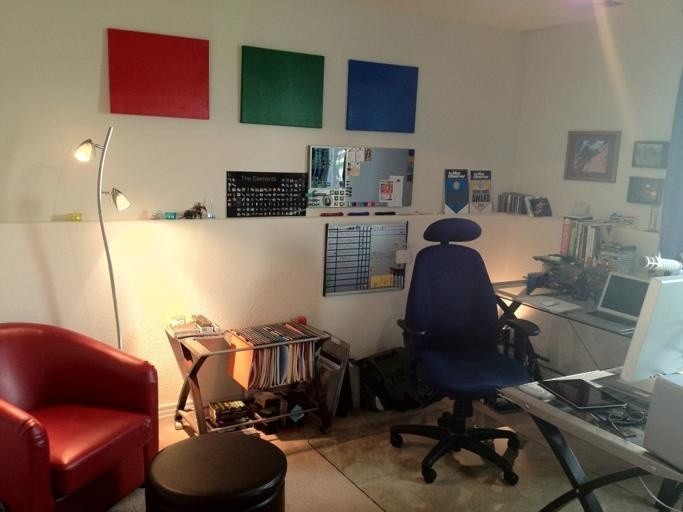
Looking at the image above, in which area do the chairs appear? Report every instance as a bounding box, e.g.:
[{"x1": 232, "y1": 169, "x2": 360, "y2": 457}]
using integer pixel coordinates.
[
  {"x1": 0, "y1": 323, "x2": 159, "y2": 512},
  {"x1": 389, "y1": 217, "x2": 540, "y2": 485}
]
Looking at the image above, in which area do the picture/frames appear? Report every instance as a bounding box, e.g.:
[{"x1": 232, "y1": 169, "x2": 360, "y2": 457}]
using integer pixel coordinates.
[
  {"x1": 632, "y1": 140, "x2": 670, "y2": 169},
  {"x1": 626, "y1": 177, "x2": 665, "y2": 206},
  {"x1": 563, "y1": 131, "x2": 621, "y2": 183}
]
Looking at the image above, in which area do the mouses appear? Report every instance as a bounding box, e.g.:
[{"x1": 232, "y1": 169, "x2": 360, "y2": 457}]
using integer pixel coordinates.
[{"x1": 542, "y1": 297, "x2": 558, "y2": 307}]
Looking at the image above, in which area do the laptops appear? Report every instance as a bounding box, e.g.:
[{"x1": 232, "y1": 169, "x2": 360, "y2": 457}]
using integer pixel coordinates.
[{"x1": 567, "y1": 272, "x2": 649, "y2": 333}]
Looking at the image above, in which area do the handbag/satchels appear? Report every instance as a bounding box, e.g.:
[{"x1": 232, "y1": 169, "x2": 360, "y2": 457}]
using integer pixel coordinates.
[{"x1": 349, "y1": 346, "x2": 446, "y2": 412}]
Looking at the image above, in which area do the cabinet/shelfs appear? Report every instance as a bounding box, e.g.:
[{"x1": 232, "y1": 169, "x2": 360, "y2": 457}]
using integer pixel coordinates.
[{"x1": 164, "y1": 318, "x2": 329, "y2": 436}]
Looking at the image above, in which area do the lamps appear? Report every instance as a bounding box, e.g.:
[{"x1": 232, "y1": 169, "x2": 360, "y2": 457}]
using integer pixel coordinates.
[{"x1": 74, "y1": 125, "x2": 130, "y2": 352}]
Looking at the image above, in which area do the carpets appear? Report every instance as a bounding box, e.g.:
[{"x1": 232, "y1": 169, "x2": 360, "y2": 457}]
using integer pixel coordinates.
[{"x1": 308, "y1": 402, "x2": 662, "y2": 512}]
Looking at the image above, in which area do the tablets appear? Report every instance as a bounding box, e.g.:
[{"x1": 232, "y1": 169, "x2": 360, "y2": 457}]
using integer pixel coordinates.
[{"x1": 538, "y1": 378, "x2": 626, "y2": 410}]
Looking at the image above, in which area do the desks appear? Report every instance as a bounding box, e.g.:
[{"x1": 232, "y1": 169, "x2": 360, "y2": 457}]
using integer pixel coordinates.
[
  {"x1": 493, "y1": 281, "x2": 636, "y2": 377},
  {"x1": 495, "y1": 366, "x2": 683, "y2": 512}
]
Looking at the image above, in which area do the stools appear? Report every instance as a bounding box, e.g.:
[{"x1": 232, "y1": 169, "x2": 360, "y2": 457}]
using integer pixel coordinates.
[{"x1": 145, "y1": 432, "x2": 287, "y2": 512}]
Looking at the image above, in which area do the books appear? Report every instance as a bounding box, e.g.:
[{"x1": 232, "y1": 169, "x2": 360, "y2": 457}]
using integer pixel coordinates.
[
  {"x1": 498, "y1": 192, "x2": 552, "y2": 217},
  {"x1": 560, "y1": 215, "x2": 615, "y2": 263}
]
